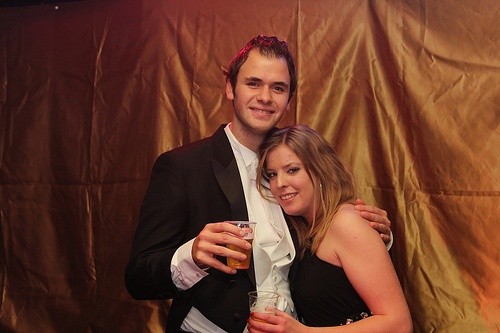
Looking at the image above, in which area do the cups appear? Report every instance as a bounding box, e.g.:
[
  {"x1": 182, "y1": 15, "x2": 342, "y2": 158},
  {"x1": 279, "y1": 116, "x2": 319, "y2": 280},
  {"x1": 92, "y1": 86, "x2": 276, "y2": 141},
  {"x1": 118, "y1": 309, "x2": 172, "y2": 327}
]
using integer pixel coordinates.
[
  {"x1": 222, "y1": 221, "x2": 258, "y2": 270},
  {"x1": 248, "y1": 290, "x2": 280, "y2": 328}
]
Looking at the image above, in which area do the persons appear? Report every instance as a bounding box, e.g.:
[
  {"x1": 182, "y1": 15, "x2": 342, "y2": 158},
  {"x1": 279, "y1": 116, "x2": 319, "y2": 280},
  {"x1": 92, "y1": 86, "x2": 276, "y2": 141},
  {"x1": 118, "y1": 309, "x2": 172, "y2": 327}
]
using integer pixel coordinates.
[
  {"x1": 242, "y1": 118, "x2": 416, "y2": 333},
  {"x1": 118, "y1": 31, "x2": 396, "y2": 333}
]
